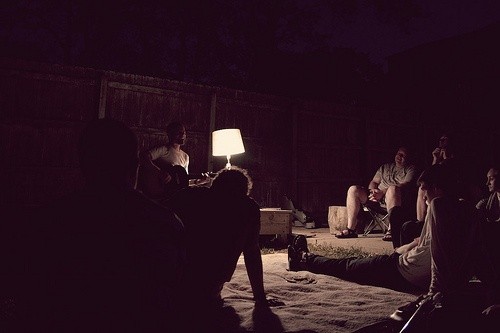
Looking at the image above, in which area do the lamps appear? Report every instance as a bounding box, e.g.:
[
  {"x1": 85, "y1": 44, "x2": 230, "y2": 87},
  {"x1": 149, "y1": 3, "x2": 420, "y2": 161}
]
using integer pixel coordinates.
[{"x1": 212, "y1": 128, "x2": 246, "y2": 170}]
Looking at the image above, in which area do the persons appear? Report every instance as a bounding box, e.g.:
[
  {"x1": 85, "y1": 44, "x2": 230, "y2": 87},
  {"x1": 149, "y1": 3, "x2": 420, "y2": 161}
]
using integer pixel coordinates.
[
  {"x1": 473, "y1": 163, "x2": 500, "y2": 289},
  {"x1": 163, "y1": 165, "x2": 285, "y2": 333},
  {"x1": 287, "y1": 166, "x2": 480, "y2": 307},
  {"x1": 144, "y1": 120, "x2": 211, "y2": 190},
  {"x1": 25, "y1": 118, "x2": 190, "y2": 333},
  {"x1": 334, "y1": 145, "x2": 422, "y2": 241},
  {"x1": 388, "y1": 142, "x2": 480, "y2": 254}
]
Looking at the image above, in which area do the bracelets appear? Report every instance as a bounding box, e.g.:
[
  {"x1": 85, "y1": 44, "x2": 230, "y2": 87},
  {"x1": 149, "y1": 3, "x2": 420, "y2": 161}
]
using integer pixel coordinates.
[{"x1": 194, "y1": 179, "x2": 199, "y2": 185}]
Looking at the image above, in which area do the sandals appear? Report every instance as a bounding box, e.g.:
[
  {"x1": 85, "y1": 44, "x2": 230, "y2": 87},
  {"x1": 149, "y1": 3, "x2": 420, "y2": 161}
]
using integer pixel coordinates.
[
  {"x1": 336, "y1": 227, "x2": 358, "y2": 238},
  {"x1": 382, "y1": 229, "x2": 393, "y2": 241}
]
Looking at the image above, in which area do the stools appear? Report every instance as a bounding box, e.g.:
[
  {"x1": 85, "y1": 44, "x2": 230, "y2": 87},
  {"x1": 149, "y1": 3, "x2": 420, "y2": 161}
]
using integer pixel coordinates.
[{"x1": 364, "y1": 205, "x2": 392, "y2": 236}]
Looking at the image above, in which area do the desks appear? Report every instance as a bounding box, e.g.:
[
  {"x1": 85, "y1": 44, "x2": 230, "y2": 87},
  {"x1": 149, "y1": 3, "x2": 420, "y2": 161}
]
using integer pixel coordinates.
[{"x1": 258, "y1": 210, "x2": 292, "y2": 242}]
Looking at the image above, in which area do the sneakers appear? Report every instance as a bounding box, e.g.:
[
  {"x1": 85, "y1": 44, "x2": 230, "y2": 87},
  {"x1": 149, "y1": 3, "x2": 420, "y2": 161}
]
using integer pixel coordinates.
[
  {"x1": 294, "y1": 234, "x2": 305, "y2": 250},
  {"x1": 288, "y1": 245, "x2": 299, "y2": 271}
]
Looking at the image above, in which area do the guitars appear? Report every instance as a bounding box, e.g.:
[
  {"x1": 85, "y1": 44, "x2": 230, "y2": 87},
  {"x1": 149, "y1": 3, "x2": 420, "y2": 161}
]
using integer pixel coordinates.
[{"x1": 140, "y1": 160, "x2": 216, "y2": 186}]
